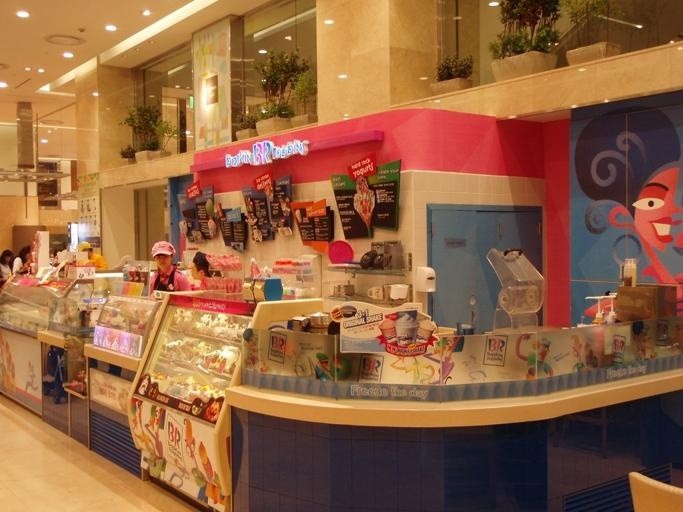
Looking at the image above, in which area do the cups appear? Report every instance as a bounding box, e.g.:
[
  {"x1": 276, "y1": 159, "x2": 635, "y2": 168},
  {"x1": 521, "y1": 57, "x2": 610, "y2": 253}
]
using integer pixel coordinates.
[
  {"x1": 366, "y1": 285, "x2": 383, "y2": 301},
  {"x1": 121, "y1": 263, "x2": 151, "y2": 283},
  {"x1": 377, "y1": 326, "x2": 432, "y2": 346},
  {"x1": 295, "y1": 209, "x2": 303, "y2": 224}
]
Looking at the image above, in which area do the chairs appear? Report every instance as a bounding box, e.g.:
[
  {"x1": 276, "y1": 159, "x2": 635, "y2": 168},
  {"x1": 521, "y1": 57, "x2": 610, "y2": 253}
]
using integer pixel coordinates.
[{"x1": 628, "y1": 472, "x2": 683, "y2": 512}]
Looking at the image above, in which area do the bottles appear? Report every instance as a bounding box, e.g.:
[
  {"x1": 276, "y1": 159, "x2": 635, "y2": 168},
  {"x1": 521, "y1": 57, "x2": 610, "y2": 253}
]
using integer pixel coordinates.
[{"x1": 333, "y1": 285, "x2": 354, "y2": 298}]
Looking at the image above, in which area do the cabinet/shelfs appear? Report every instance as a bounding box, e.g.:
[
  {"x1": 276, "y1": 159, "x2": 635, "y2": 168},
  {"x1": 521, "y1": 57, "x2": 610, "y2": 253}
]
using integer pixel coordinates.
[{"x1": 325, "y1": 267, "x2": 409, "y2": 305}]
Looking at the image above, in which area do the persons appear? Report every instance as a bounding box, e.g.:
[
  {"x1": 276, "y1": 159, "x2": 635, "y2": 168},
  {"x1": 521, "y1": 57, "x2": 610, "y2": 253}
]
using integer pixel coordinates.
[
  {"x1": 190, "y1": 251, "x2": 221, "y2": 280},
  {"x1": 0, "y1": 249, "x2": 13, "y2": 288},
  {"x1": 78, "y1": 241, "x2": 105, "y2": 269},
  {"x1": 11, "y1": 245, "x2": 31, "y2": 274},
  {"x1": 148, "y1": 240, "x2": 193, "y2": 296}
]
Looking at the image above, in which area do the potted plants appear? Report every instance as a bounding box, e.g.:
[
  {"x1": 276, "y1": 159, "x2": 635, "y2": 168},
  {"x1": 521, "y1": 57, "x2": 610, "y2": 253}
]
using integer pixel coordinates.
[
  {"x1": 118, "y1": 104, "x2": 191, "y2": 166},
  {"x1": 233, "y1": 44, "x2": 317, "y2": 140},
  {"x1": 429, "y1": 0, "x2": 626, "y2": 96}
]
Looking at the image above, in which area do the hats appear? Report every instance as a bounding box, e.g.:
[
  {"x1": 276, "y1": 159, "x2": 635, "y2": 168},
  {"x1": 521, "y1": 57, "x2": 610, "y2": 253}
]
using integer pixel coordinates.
[
  {"x1": 151, "y1": 241, "x2": 176, "y2": 258},
  {"x1": 77, "y1": 242, "x2": 92, "y2": 252}
]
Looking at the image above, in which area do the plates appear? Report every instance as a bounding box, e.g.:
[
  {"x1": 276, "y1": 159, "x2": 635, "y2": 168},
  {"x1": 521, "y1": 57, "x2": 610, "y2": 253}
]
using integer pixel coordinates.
[{"x1": 329, "y1": 240, "x2": 353, "y2": 265}]
